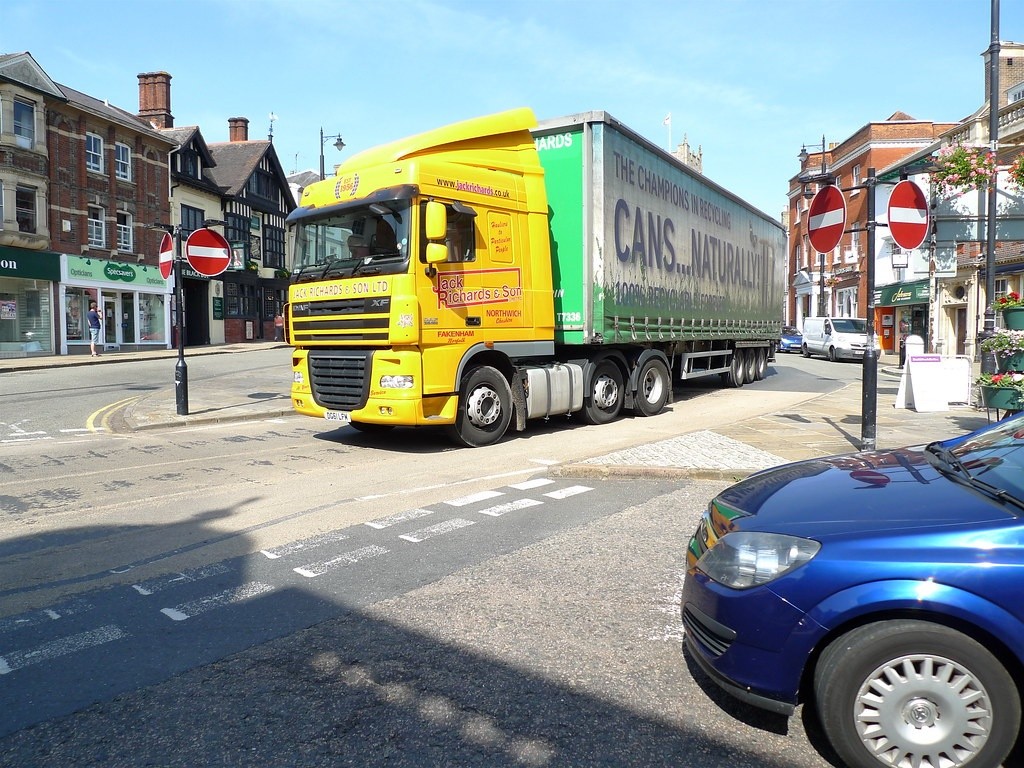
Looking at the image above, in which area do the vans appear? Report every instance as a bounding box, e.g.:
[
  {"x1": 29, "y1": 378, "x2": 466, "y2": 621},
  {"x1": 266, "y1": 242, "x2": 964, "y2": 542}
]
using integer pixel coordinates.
[{"x1": 801, "y1": 316, "x2": 882, "y2": 361}]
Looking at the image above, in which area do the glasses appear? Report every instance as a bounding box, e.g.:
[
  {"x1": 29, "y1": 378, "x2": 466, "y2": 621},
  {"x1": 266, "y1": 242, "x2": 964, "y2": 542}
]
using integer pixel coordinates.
[{"x1": 96, "y1": 305, "x2": 97, "y2": 307}]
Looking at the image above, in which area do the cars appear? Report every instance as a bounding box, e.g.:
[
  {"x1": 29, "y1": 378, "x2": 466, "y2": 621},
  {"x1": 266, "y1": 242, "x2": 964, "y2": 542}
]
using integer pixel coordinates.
[
  {"x1": 678, "y1": 408, "x2": 1024, "y2": 768},
  {"x1": 775, "y1": 326, "x2": 802, "y2": 353}
]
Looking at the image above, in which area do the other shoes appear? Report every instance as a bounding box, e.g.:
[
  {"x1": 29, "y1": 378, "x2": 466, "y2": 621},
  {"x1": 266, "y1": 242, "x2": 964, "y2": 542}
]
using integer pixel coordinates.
[{"x1": 92, "y1": 353, "x2": 101, "y2": 357}]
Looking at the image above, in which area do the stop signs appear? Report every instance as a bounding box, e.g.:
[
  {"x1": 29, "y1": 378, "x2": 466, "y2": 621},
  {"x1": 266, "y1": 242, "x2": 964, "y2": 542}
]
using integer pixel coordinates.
[
  {"x1": 806, "y1": 185, "x2": 846, "y2": 256},
  {"x1": 185, "y1": 227, "x2": 232, "y2": 276},
  {"x1": 159, "y1": 234, "x2": 171, "y2": 279},
  {"x1": 886, "y1": 180, "x2": 930, "y2": 249}
]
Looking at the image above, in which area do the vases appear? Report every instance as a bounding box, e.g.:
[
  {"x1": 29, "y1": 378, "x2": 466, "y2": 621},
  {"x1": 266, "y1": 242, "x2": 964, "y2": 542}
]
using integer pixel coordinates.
[
  {"x1": 981, "y1": 386, "x2": 1024, "y2": 410},
  {"x1": 997, "y1": 350, "x2": 1024, "y2": 371},
  {"x1": 1001, "y1": 306, "x2": 1024, "y2": 330}
]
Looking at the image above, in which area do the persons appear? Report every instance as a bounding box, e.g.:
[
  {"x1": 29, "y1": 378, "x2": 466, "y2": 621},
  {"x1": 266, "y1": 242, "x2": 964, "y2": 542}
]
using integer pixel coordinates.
[
  {"x1": 347, "y1": 235, "x2": 369, "y2": 257},
  {"x1": 87, "y1": 302, "x2": 102, "y2": 357},
  {"x1": 274, "y1": 313, "x2": 284, "y2": 341}
]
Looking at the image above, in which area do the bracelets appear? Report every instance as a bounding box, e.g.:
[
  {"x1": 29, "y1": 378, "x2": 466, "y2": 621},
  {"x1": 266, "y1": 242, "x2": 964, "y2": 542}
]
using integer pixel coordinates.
[{"x1": 98, "y1": 310, "x2": 101, "y2": 312}]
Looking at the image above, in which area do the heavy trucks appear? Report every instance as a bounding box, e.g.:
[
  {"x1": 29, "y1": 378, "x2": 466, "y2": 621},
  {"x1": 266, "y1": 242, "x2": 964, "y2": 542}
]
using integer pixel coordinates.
[{"x1": 284, "y1": 107, "x2": 789, "y2": 449}]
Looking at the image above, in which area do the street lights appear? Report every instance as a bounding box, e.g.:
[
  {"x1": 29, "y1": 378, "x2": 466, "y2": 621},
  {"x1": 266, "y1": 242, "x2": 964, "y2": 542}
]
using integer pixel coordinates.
[
  {"x1": 319, "y1": 126, "x2": 347, "y2": 182},
  {"x1": 798, "y1": 134, "x2": 826, "y2": 173}
]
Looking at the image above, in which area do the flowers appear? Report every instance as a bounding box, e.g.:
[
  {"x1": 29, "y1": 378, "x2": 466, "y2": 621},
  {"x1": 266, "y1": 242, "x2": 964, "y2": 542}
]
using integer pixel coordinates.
[
  {"x1": 975, "y1": 371, "x2": 1024, "y2": 403},
  {"x1": 989, "y1": 291, "x2": 1024, "y2": 311},
  {"x1": 922, "y1": 146, "x2": 1003, "y2": 201},
  {"x1": 979, "y1": 330, "x2": 1024, "y2": 359},
  {"x1": 1004, "y1": 151, "x2": 1024, "y2": 193}
]
